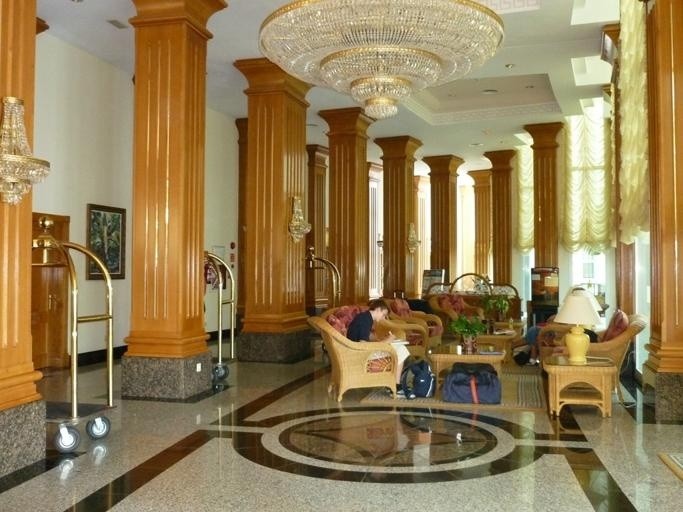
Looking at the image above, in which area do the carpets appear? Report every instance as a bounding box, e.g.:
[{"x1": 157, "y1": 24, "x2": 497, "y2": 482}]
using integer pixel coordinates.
[
  {"x1": 360, "y1": 338, "x2": 547, "y2": 409},
  {"x1": 658, "y1": 451, "x2": 683, "y2": 481}
]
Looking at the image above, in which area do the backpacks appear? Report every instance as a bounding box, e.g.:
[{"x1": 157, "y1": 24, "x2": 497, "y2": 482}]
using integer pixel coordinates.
[{"x1": 401, "y1": 356, "x2": 435, "y2": 399}]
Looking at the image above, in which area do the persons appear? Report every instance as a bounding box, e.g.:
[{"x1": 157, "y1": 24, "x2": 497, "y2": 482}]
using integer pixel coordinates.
[
  {"x1": 512, "y1": 288, "x2": 585, "y2": 366},
  {"x1": 346, "y1": 300, "x2": 416, "y2": 399}
]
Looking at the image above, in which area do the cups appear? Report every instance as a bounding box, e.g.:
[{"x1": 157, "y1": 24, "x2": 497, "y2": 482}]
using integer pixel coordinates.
[
  {"x1": 456, "y1": 344, "x2": 463, "y2": 355},
  {"x1": 488, "y1": 346, "x2": 495, "y2": 352}
]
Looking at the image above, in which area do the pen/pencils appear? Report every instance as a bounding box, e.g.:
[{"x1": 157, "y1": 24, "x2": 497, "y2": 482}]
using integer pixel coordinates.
[{"x1": 389, "y1": 331, "x2": 393, "y2": 336}]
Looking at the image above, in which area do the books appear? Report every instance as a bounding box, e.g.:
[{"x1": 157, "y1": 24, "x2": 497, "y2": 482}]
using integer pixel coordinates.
[
  {"x1": 495, "y1": 328, "x2": 515, "y2": 335},
  {"x1": 391, "y1": 339, "x2": 409, "y2": 345}
]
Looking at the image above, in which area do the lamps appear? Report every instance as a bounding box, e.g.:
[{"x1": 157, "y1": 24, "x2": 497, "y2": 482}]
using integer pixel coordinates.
[
  {"x1": 407, "y1": 223, "x2": 421, "y2": 252},
  {"x1": 288, "y1": 197, "x2": 312, "y2": 243},
  {"x1": 553, "y1": 290, "x2": 604, "y2": 362},
  {"x1": 257, "y1": 0, "x2": 505, "y2": 120},
  {"x1": 0, "y1": 96, "x2": 50, "y2": 206}
]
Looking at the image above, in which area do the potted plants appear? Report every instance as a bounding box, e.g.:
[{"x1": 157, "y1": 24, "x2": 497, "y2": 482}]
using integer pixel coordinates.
[{"x1": 484, "y1": 291, "x2": 517, "y2": 321}]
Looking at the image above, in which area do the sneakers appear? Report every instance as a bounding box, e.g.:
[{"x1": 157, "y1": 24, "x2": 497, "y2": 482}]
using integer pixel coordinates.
[{"x1": 526, "y1": 362, "x2": 538, "y2": 365}]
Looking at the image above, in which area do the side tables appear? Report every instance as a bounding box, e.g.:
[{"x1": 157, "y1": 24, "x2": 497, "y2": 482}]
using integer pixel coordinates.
[{"x1": 543, "y1": 354, "x2": 618, "y2": 418}]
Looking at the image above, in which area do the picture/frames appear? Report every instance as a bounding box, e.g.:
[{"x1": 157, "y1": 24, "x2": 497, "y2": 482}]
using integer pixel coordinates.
[{"x1": 86, "y1": 204, "x2": 126, "y2": 280}]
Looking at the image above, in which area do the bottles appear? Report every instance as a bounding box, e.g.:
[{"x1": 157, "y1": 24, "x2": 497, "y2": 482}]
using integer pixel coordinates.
[{"x1": 509, "y1": 317, "x2": 514, "y2": 330}]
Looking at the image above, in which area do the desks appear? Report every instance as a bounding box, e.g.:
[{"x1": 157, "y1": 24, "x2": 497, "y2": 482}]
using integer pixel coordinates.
[
  {"x1": 477, "y1": 331, "x2": 518, "y2": 363},
  {"x1": 426, "y1": 346, "x2": 506, "y2": 391},
  {"x1": 493, "y1": 321, "x2": 524, "y2": 343}
]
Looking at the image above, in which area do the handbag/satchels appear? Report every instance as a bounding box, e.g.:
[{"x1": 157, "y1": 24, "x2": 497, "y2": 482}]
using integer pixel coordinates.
[
  {"x1": 513, "y1": 350, "x2": 528, "y2": 367},
  {"x1": 440, "y1": 363, "x2": 502, "y2": 404}
]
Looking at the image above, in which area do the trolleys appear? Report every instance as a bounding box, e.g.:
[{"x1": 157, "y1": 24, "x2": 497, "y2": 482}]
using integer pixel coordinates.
[
  {"x1": 31, "y1": 216, "x2": 118, "y2": 453},
  {"x1": 305, "y1": 245, "x2": 343, "y2": 353},
  {"x1": 203, "y1": 250, "x2": 238, "y2": 386}
]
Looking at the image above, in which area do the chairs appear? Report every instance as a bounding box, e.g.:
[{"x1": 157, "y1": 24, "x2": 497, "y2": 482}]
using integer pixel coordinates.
[
  {"x1": 537, "y1": 303, "x2": 647, "y2": 402},
  {"x1": 306, "y1": 294, "x2": 484, "y2": 402}
]
